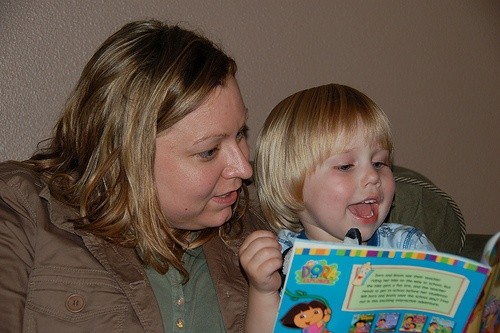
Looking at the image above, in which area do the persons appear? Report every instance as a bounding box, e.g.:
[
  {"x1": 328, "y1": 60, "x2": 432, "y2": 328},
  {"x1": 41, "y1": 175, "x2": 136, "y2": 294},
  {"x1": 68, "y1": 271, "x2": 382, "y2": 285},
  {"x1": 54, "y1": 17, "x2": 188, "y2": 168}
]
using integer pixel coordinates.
[
  {"x1": 0, "y1": 18, "x2": 271, "y2": 333},
  {"x1": 239, "y1": 84, "x2": 439, "y2": 333}
]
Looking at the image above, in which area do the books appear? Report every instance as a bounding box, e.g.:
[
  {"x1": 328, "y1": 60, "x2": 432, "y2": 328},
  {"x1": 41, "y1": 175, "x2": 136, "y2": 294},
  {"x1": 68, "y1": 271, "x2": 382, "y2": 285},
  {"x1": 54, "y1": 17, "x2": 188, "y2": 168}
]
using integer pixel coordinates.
[{"x1": 272, "y1": 230, "x2": 500, "y2": 333}]
